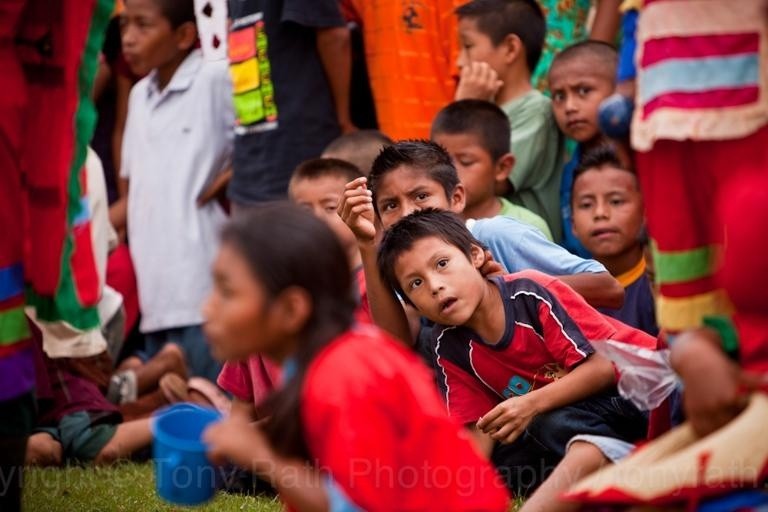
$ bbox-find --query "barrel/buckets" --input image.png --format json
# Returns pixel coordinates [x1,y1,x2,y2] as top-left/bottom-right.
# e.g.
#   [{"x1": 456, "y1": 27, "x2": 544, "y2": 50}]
[{"x1": 150, "y1": 401, "x2": 225, "y2": 506}]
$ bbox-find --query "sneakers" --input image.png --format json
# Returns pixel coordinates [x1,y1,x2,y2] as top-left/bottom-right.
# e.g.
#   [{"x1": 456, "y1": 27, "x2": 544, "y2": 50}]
[{"x1": 107, "y1": 373, "x2": 131, "y2": 406}]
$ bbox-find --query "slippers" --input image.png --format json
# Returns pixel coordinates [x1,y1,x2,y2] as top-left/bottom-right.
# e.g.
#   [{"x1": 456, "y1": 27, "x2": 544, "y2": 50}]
[{"x1": 159, "y1": 371, "x2": 235, "y2": 418}]
